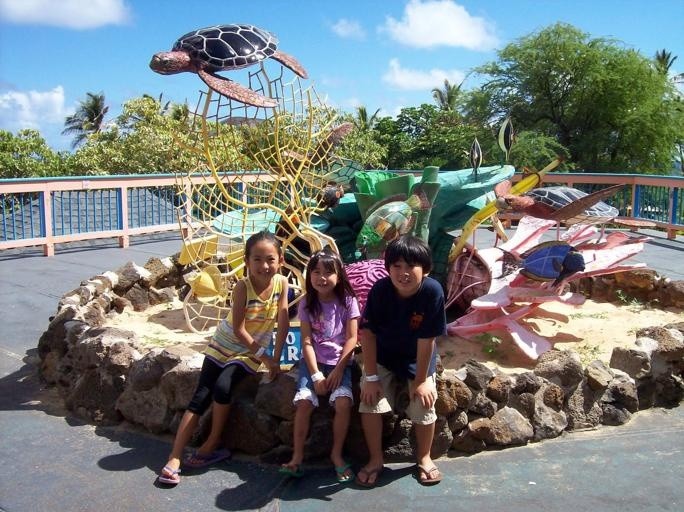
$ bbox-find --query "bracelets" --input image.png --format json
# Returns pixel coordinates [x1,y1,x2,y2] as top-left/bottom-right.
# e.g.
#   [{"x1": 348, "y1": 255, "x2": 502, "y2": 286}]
[
  {"x1": 255, "y1": 346, "x2": 266, "y2": 358},
  {"x1": 364, "y1": 374, "x2": 380, "y2": 383},
  {"x1": 310, "y1": 370, "x2": 325, "y2": 382}
]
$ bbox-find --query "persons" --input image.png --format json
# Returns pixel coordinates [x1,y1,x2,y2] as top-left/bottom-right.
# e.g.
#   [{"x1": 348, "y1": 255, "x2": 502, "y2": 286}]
[
  {"x1": 278, "y1": 251, "x2": 364, "y2": 483},
  {"x1": 356, "y1": 234, "x2": 448, "y2": 488},
  {"x1": 155, "y1": 232, "x2": 290, "y2": 483}
]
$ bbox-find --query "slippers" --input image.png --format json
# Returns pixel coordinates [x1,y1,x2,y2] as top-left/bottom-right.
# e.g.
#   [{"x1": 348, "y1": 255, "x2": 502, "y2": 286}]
[
  {"x1": 417, "y1": 465, "x2": 441, "y2": 483},
  {"x1": 159, "y1": 466, "x2": 181, "y2": 484},
  {"x1": 184, "y1": 448, "x2": 231, "y2": 467},
  {"x1": 335, "y1": 464, "x2": 383, "y2": 487},
  {"x1": 279, "y1": 464, "x2": 304, "y2": 477}
]
